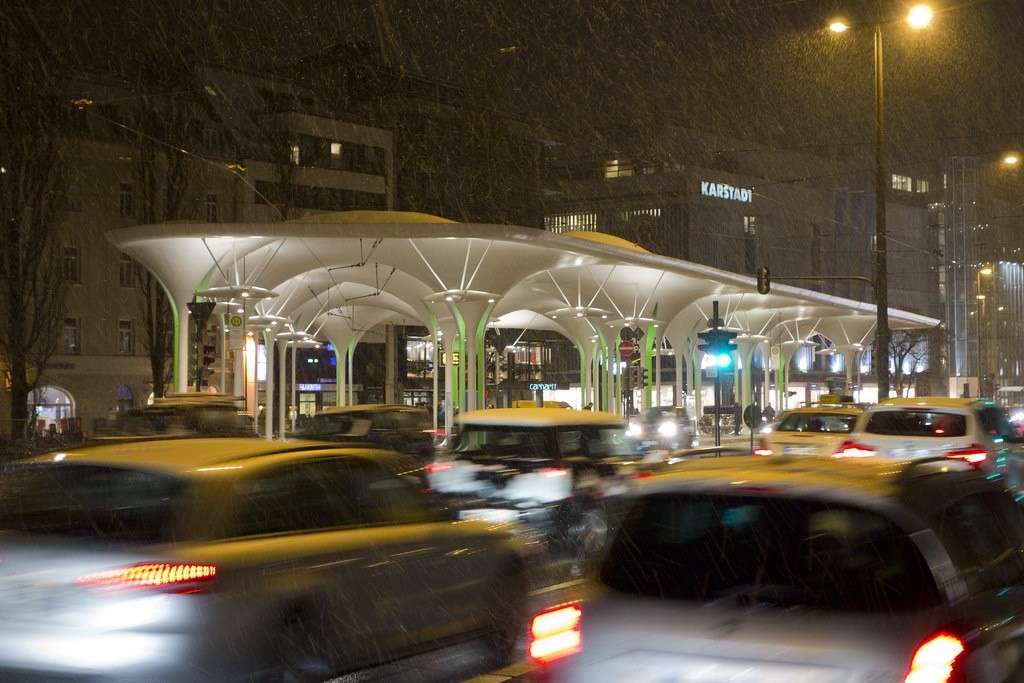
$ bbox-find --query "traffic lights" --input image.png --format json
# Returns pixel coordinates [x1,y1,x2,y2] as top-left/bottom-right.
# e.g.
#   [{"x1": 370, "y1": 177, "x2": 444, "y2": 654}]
[
  {"x1": 639, "y1": 367, "x2": 648, "y2": 388},
  {"x1": 199, "y1": 345, "x2": 216, "y2": 378},
  {"x1": 723, "y1": 331, "x2": 738, "y2": 351},
  {"x1": 716, "y1": 353, "x2": 732, "y2": 369},
  {"x1": 697, "y1": 332, "x2": 714, "y2": 354}
]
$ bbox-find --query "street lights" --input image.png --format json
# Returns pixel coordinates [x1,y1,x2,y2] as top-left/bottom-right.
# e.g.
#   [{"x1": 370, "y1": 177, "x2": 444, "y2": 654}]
[
  {"x1": 977, "y1": 265, "x2": 993, "y2": 398},
  {"x1": 827, "y1": 8, "x2": 935, "y2": 402}
]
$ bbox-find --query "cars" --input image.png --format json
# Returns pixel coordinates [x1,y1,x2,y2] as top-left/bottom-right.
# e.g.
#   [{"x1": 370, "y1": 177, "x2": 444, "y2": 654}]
[
  {"x1": 286, "y1": 404, "x2": 435, "y2": 459},
  {"x1": 524, "y1": 458, "x2": 1024, "y2": 683},
  {"x1": 828, "y1": 396, "x2": 1024, "y2": 494},
  {"x1": 627, "y1": 406, "x2": 693, "y2": 451},
  {"x1": 753, "y1": 394, "x2": 870, "y2": 458},
  {"x1": 113, "y1": 395, "x2": 259, "y2": 439},
  {"x1": 427, "y1": 406, "x2": 636, "y2": 527},
  {"x1": 0, "y1": 437, "x2": 548, "y2": 683}
]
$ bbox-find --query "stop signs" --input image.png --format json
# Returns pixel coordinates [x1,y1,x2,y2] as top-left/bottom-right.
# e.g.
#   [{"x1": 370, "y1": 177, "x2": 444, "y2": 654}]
[{"x1": 618, "y1": 341, "x2": 634, "y2": 356}]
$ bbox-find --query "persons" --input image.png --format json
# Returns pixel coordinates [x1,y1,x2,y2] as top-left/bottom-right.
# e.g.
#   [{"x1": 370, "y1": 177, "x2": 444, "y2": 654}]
[
  {"x1": 582, "y1": 401, "x2": 593, "y2": 411},
  {"x1": 734, "y1": 401, "x2": 776, "y2": 427},
  {"x1": 633, "y1": 407, "x2": 640, "y2": 415},
  {"x1": 415, "y1": 399, "x2": 495, "y2": 428}
]
